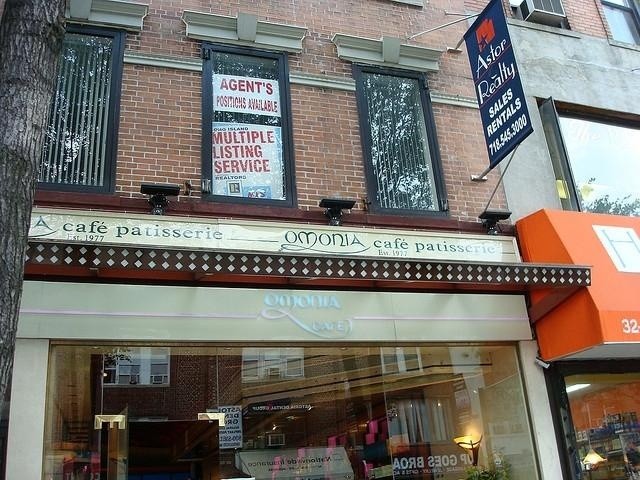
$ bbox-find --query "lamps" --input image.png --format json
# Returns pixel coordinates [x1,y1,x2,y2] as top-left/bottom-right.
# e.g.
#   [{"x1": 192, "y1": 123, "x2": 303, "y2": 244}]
[
  {"x1": 582, "y1": 415, "x2": 608, "y2": 468},
  {"x1": 456, "y1": 431, "x2": 487, "y2": 464},
  {"x1": 482, "y1": 210, "x2": 512, "y2": 236},
  {"x1": 141, "y1": 178, "x2": 182, "y2": 215},
  {"x1": 317, "y1": 195, "x2": 352, "y2": 225}
]
